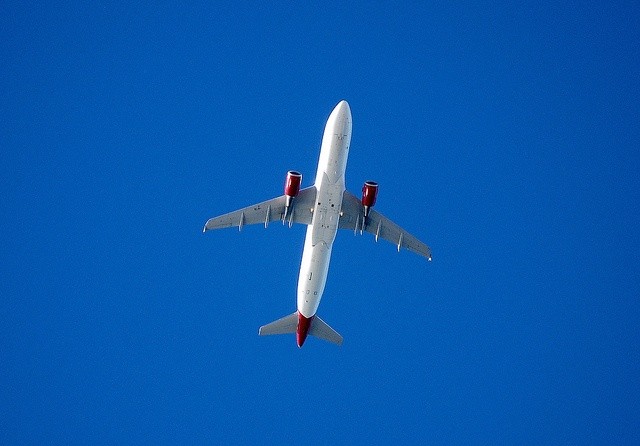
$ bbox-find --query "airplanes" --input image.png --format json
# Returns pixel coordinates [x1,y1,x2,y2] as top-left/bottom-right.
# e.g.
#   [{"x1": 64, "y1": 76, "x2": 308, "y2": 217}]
[{"x1": 203, "y1": 100, "x2": 431, "y2": 349}]
